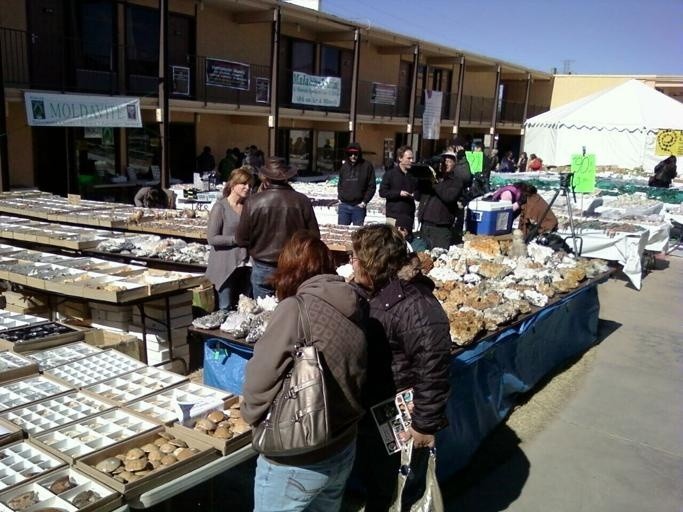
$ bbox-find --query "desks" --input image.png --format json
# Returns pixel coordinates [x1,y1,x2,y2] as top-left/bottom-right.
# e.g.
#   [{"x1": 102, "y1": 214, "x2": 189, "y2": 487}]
[{"x1": 1, "y1": 171, "x2": 682, "y2": 512}]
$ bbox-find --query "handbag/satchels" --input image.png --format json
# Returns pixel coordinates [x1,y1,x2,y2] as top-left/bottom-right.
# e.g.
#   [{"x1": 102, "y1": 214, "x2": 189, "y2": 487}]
[
  {"x1": 648, "y1": 176, "x2": 656, "y2": 187},
  {"x1": 459, "y1": 176, "x2": 485, "y2": 206},
  {"x1": 384, "y1": 435, "x2": 445, "y2": 512},
  {"x1": 249, "y1": 295, "x2": 332, "y2": 458},
  {"x1": 536, "y1": 232, "x2": 572, "y2": 254}
]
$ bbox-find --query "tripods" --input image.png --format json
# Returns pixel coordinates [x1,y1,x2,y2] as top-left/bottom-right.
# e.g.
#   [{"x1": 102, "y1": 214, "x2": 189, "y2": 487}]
[{"x1": 524, "y1": 173, "x2": 579, "y2": 258}]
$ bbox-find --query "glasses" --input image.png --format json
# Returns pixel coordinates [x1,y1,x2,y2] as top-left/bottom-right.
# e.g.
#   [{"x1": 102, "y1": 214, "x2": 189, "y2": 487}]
[
  {"x1": 347, "y1": 152, "x2": 358, "y2": 157},
  {"x1": 396, "y1": 227, "x2": 408, "y2": 231},
  {"x1": 348, "y1": 254, "x2": 359, "y2": 263}
]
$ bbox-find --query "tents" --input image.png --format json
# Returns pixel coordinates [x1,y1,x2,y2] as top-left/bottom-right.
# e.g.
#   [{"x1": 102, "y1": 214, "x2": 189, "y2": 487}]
[{"x1": 519, "y1": 79, "x2": 682, "y2": 170}]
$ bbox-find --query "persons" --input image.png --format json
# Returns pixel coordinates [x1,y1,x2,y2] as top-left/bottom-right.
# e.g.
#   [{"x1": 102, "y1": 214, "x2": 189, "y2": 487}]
[
  {"x1": 240, "y1": 232, "x2": 370, "y2": 512},
  {"x1": 347, "y1": 221, "x2": 455, "y2": 512},
  {"x1": 134, "y1": 186, "x2": 169, "y2": 208},
  {"x1": 652, "y1": 156, "x2": 677, "y2": 185}
]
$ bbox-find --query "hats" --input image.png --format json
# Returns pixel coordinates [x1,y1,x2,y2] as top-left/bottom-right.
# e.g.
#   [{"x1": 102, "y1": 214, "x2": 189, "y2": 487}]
[
  {"x1": 394, "y1": 213, "x2": 413, "y2": 230},
  {"x1": 258, "y1": 156, "x2": 297, "y2": 181},
  {"x1": 441, "y1": 150, "x2": 458, "y2": 163}
]
